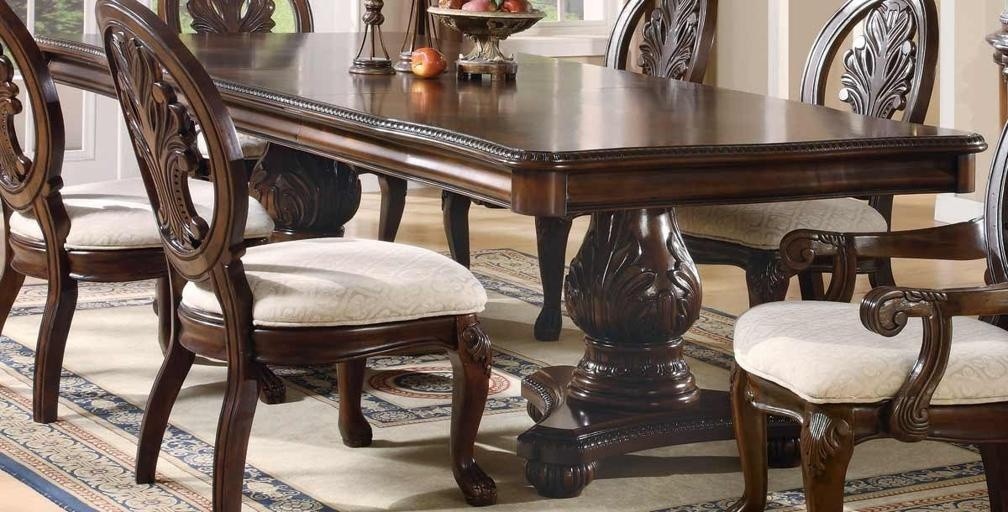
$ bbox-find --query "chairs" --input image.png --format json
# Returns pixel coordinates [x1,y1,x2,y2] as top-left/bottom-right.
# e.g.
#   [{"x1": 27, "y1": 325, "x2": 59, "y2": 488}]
[
  {"x1": 440, "y1": 1, "x2": 719, "y2": 342},
  {"x1": 731, "y1": 113, "x2": 1008, "y2": 512},
  {"x1": 156, "y1": 0, "x2": 407, "y2": 241},
  {"x1": 96, "y1": 0, "x2": 498, "y2": 512},
  {"x1": 675, "y1": 1, "x2": 940, "y2": 314},
  {"x1": 0, "y1": 0, "x2": 286, "y2": 424}
]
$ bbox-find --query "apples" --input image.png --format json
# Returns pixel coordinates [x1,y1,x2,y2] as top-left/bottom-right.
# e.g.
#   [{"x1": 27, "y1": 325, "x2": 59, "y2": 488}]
[
  {"x1": 412, "y1": 79, "x2": 444, "y2": 107},
  {"x1": 501, "y1": 0, "x2": 530, "y2": 13},
  {"x1": 461, "y1": 1, "x2": 496, "y2": 11},
  {"x1": 438, "y1": 0, "x2": 462, "y2": 9},
  {"x1": 411, "y1": 48, "x2": 448, "y2": 78}
]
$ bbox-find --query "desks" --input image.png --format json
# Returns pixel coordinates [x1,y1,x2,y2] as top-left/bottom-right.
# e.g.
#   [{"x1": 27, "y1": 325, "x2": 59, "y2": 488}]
[{"x1": 28, "y1": 30, "x2": 988, "y2": 498}]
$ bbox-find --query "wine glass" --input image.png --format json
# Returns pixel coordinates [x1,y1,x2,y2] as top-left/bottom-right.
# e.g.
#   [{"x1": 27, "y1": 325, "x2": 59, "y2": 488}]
[{"x1": 426, "y1": 5, "x2": 546, "y2": 81}]
